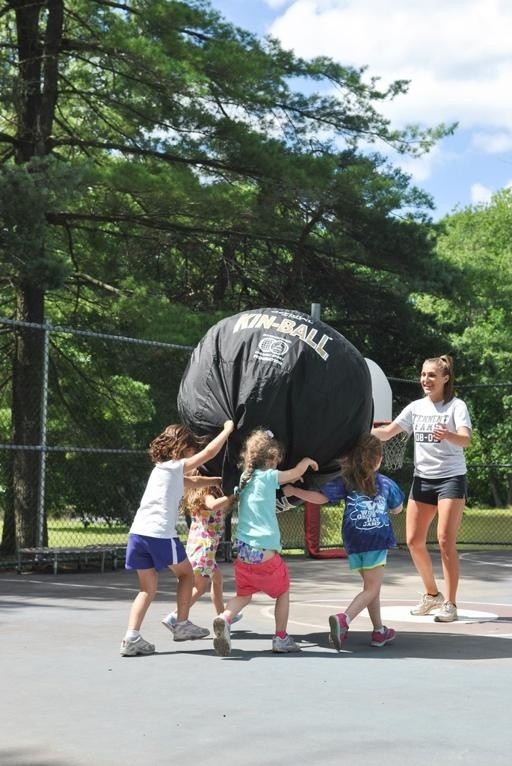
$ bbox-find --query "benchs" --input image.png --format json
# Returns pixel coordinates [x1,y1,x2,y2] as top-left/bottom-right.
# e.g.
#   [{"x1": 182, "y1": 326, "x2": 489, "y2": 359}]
[{"x1": 16, "y1": 547, "x2": 125, "y2": 576}]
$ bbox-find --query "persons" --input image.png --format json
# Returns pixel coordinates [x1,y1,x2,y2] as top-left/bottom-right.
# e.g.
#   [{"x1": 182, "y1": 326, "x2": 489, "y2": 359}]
[
  {"x1": 370, "y1": 355, "x2": 473, "y2": 624},
  {"x1": 211, "y1": 426, "x2": 320, "y2": 660},
  {"x1": 161, "y1": 465, "x2": 243, "y2": 635},
  {"x1": 280, "y1": 432, "x2": 405, "y2": 648},
  {"x1": 118, "y1": 418, "x2": 236, "y2": 657}
]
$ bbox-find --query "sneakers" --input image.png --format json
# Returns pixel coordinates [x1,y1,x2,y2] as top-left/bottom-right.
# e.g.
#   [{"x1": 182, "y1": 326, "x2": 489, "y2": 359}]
[
  {"x1": 372, "y1": 625, "x2": 396, "y2": 646},
  {"x1": 162, "y1": 612, "x2": 178, "y2": 633},
  {"x1": 329, "y1": 613, "x2": 349, "y2": 652},
  {"x1": 231, "y1": 613, "x2": 243, "y2": 624},
  {"x1": 172, "y1": 620, "x2": 211, "y2": 640},
  {"x1": 434, "y1": 602, "x2": 458, "y2": 622},
  {"x1": 119, "y1": 634, "x2": 156, "y2": 657},
  {"x1": 410, "y1": 592, "x2": 446, "y2": 616},
  {"x1": 273, "y1": 633, "x2": 302, "y2": 654},
  {"x1": 213, "y1": 616, "x2": 231, "y2": 657}
]
